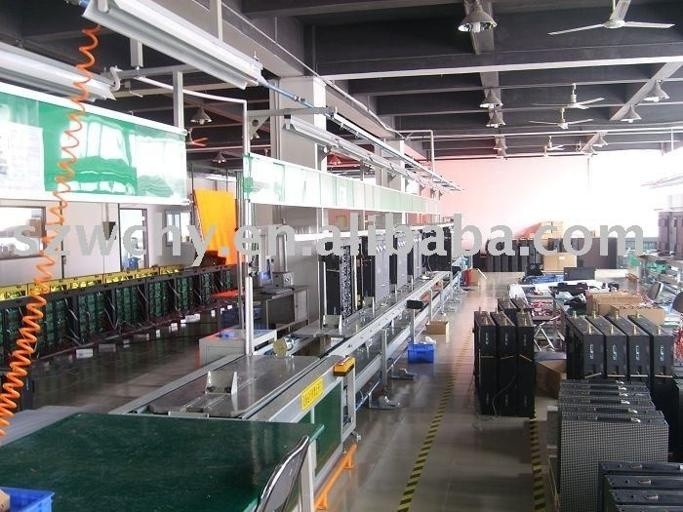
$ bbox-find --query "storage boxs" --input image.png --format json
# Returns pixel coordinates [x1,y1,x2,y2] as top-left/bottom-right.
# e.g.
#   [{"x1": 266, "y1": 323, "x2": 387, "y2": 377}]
[
  {"x1": 611, "y1": 305, "x2": 666, "y2": 324},
  {"x1": 535, "y1": 359, "x2": 567, "y2": 401},
  {"x1": 425, "y1": 318, "x2": 450, "y2": 335},
  {"x1": 0, "y1": 487, "x2": 54, "y2": 512},
  {"x1": 408, "y1": 344, "x2": 434, "y2": 363},
  {"x1": 542, "y1": 252, "x2": 577, "y2": 271},
  {"x1": 27, "y1": 219, "x2": 43, "y2": 238},
  {"x1": 587, "y1": 293, "x2": 642, "y2": 315},
  {"x1": 462, "y1": 267, "x2": 481, "y2": 287}
]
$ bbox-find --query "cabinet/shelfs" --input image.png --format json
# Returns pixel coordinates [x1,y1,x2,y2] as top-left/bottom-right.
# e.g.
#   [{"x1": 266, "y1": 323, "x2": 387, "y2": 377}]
[
  {"x1": 200, "y1": 329, "x2": 277, "y2": 365},
  {"x1": 198, "y1": 284, "x2": 306, "y2": 332}
]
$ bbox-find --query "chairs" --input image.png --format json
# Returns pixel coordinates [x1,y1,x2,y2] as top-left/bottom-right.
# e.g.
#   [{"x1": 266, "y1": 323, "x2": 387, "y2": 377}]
[{"x1": 254, "y1": 434, "x2": 310, "y2": 511}]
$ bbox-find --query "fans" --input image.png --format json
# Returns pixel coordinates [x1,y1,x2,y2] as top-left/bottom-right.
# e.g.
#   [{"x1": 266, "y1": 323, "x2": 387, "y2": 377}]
[
  {"x1": 532, "y1": 96, "x2": 605, "y2": 110},
  {"x1": 547, "y1": 144, "x2": 566, "y2": 151},
  {"x1": 528, "y1": 118, "x2": 594, "y2": 130},
  {"x1": 187, "y1": 130, "x2": 207, "y2": 148},
  {"x1": 546, "y1": 0, "x2": 676, "y2": 37}
]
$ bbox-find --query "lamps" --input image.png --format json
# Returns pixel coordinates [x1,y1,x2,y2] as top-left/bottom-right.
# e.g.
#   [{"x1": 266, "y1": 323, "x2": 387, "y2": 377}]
[
  {"x1": 492, "y1": 139, "x2": 508, "y2": 151},
  {"x1": 479, "y1": 88, "x2": 504, "y2": 110},
  {"x1": 279, "y1": 115, "x2": 339, "y2": 156},
  {"x1": 338, "y1": 133, "x2": 368, "y2": 167},
  {"x1": 368, "y1": 150, "x2": 392, "y2": 172},
  {"x1": 485, "y1": 110, "x2": 507, "y2": 129},
  {"x1": 643, "y1": 80, "x2": 670, "y2": 103},
  {"x1": 212, "y1": 152, "x2": 228, "y2": 164},
  {"x1": 496, "y1": 150, "x2": 508, "y2": 160},
  {"x1": 582, "y1": 145, "x2": 598, "y2": 158},
  {"x1": 592, "y1": 134, "x2": 608, "y2": 148},
  {"x1": 81, "y1": 0, "x2": 264, "y2": 91},
  {"x1": 458, "y1": 0, "x2": 497, "y2": 34},
  {"x1": 394, "y1": 162, "x2": 450, "y2": 198},
  {"x1": 620, "y1": 104, "x2": 643, "y2": 124},
  {"x1": 190, "y1": 106, "x2": 212, "y2": 125}
]
